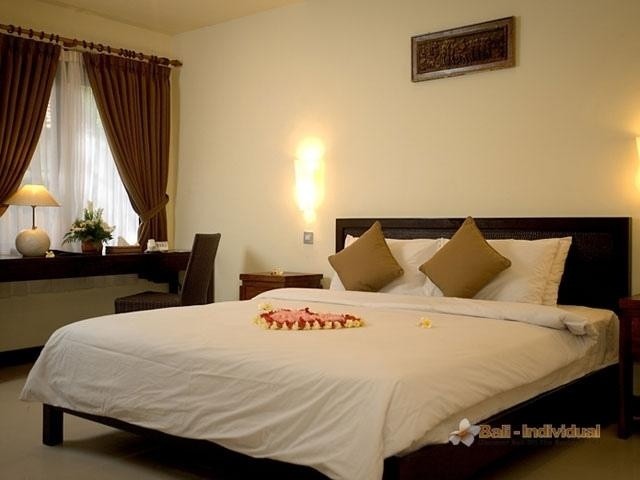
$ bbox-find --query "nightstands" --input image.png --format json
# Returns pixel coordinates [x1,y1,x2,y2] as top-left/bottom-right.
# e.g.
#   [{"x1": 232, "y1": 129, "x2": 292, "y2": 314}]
[{"x1": 236, "y1": 269, "x2": 324, "y2": 304}]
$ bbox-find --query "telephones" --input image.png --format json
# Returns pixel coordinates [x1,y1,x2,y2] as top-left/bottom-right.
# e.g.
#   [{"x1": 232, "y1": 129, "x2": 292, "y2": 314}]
[{"x1": 147, "y1": 239, "x2": 169, "y2": 252}]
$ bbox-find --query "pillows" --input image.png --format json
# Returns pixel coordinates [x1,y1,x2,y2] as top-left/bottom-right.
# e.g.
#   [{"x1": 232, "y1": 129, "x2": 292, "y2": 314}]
[
  {"x1": 327, "y1": 220, "x2": 404, "y2": 294},
  {"x1": 328, "y1": 234, "x2": 438, "y2": 298},
  {"x1": 427, "y1": 236, "x2": 574, "y2": 308},
  {"x1": 417, "y1": 215, "x2": 513, "y2": 299}
]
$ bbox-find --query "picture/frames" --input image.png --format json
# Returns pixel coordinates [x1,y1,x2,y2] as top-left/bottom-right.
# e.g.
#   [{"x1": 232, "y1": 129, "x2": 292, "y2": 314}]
[{"x1": 409, "y1": 16, "x2": 518, "y2": 85}]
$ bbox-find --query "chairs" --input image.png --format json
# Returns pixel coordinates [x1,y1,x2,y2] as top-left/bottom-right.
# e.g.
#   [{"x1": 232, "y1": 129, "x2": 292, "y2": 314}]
[{"x1": 114, "y1": 232, "x2": 224, "y2": 314}]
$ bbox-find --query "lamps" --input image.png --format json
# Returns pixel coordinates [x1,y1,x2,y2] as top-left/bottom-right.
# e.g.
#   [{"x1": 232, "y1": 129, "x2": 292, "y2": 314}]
[{"x1": 1, "y1": 182, "x2": 62, "y2": 257}]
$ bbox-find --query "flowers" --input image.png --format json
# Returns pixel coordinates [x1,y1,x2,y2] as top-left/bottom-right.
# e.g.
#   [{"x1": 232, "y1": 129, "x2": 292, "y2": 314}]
[
  {"x1": 413, "y1": 315, "x2": 433, "y2": 330},
  {"x1": 253, "y1": 303, "x2": 367, "y2": 332},
  {"x1": 62, "y1": 198, "x2": 118, "y2": 245}
]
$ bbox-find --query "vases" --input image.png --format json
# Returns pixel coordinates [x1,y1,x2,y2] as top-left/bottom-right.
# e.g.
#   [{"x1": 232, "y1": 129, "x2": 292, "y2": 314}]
[{"x1": 81, "y1": 238, "x2": 104, "y2": 254}]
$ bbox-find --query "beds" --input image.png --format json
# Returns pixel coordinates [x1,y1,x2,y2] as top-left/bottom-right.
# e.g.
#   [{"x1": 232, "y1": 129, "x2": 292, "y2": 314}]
[{"x1": 19, "y1": 211, "x2": 639, "y2": 480}]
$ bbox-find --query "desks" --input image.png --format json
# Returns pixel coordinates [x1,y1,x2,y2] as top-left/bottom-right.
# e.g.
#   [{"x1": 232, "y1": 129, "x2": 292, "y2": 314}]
[
  {"x1": 616, "y1": 288, "x2": 640, "y2": 438},
  {"x1": 0, "y1": 247, "x2": 217, "y2": 330}
]
